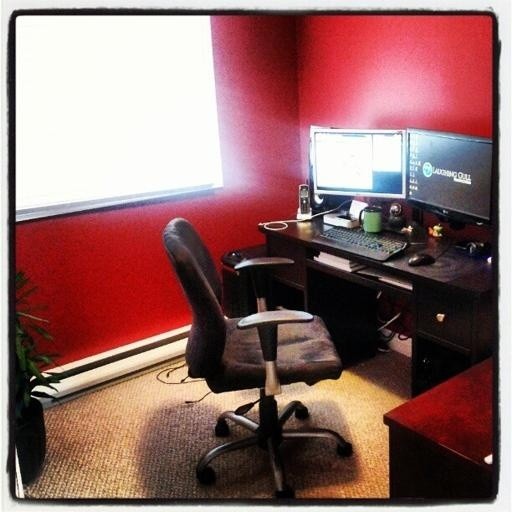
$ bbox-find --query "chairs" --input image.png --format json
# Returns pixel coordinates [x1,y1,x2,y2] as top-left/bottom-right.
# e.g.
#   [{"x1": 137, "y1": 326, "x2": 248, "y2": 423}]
[{"x1": 161, "y1": 217, "x2": 353, "y2": 499}]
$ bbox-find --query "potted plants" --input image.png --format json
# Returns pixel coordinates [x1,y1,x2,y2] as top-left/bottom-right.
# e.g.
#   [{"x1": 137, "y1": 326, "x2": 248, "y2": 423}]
[{"x1": 13, "y1": 271, "x2": 66, "y2": 485}]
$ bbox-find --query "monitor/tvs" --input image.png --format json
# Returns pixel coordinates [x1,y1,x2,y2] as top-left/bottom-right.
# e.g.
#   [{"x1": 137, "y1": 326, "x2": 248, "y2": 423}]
[
  {"x1": 313, "y1": 129, "x2": 406, "y2": 199},
  {"x1": 406, "y1": 128, "x2": 493, "y2": 224}
]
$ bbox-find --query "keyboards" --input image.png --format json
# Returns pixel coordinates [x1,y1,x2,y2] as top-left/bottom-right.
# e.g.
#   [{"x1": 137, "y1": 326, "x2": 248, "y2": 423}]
[{"x1": 312, "y1": 226, "x2": 408, "y2": 261}]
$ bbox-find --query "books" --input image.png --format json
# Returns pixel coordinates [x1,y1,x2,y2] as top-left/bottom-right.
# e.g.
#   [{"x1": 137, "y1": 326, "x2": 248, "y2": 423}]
[{"x1": 313, "y1": 251, "x2": 368, "y2": 273}]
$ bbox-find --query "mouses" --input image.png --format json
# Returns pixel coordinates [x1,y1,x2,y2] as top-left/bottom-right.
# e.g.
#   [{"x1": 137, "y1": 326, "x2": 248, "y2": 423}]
[{"x1": 409, "y1": 253, "x2": 435, "y2": 267}]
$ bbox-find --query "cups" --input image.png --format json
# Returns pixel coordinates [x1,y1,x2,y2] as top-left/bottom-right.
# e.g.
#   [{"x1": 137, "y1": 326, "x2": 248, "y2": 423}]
[{"x1": 359, "y1": 206, "x2": 384, "y2": 233}]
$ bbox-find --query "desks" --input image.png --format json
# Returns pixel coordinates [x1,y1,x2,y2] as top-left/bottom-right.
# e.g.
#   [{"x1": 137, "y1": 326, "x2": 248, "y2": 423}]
[{"x1": 258, "y1": 218, "x2": 493, "y2": 398}]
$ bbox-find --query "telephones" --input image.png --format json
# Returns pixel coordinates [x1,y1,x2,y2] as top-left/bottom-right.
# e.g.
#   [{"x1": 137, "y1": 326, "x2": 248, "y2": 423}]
[{"x1": 296, "y1": 184, "x2": 312, "y2": 220}]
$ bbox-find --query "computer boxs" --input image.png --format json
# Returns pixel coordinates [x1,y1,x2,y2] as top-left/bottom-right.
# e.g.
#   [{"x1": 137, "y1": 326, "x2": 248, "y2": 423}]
[{"x1": 220, "y1": 244, "x2": 275, "y2": 318}]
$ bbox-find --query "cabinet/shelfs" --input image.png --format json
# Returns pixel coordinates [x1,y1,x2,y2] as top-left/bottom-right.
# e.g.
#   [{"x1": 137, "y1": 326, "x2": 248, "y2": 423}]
[{"x1": 383, "y1": 356, "x2": 493, "y2": 498}]
